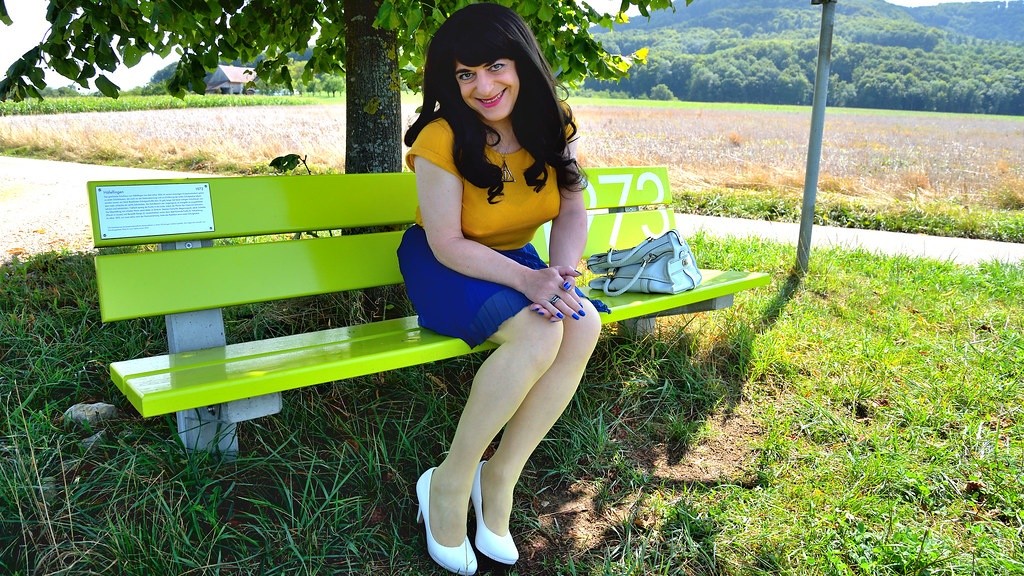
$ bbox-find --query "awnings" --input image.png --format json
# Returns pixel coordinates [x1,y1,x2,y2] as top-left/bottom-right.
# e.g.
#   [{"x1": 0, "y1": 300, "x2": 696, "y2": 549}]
[{"x1": 207, "y1": 80, "x2": 228, "y2": 89}]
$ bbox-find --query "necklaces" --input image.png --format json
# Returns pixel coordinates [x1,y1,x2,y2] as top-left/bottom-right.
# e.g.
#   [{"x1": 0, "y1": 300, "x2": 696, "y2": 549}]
[{"x1": 491, "y1": 132, "x2": 516, "y2": 182}]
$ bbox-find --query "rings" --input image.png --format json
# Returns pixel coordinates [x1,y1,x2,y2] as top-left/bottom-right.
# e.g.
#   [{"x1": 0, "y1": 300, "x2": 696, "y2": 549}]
[{"x1": 550, "y1": 295, "x2": 559, "y2": 306}]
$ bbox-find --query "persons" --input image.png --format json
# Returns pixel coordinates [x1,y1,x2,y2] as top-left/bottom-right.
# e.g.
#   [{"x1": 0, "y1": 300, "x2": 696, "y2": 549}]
[{"x1": 397, "y1": 2, "x2": 610, "y2": 574}]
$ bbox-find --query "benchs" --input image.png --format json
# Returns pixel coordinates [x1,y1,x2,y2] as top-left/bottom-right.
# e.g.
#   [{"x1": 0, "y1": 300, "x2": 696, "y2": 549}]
[{"x1": 87, "y1": 167, "x2": 769, "y2": 507}]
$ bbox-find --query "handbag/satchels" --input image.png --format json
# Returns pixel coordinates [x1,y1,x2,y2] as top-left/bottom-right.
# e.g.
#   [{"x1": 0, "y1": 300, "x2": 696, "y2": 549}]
[{"x1": 586, "y1": 229, "x2": 701, "y2": 297}]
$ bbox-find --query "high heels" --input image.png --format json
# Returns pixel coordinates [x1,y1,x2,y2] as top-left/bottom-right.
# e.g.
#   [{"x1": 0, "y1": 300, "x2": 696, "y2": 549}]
[
  {"x1": 466, "y1": 460, "x2": 519, "y2": 565},
  {"x1": 416, "y1": 468, "x2": 478, "y2": 576}
]
